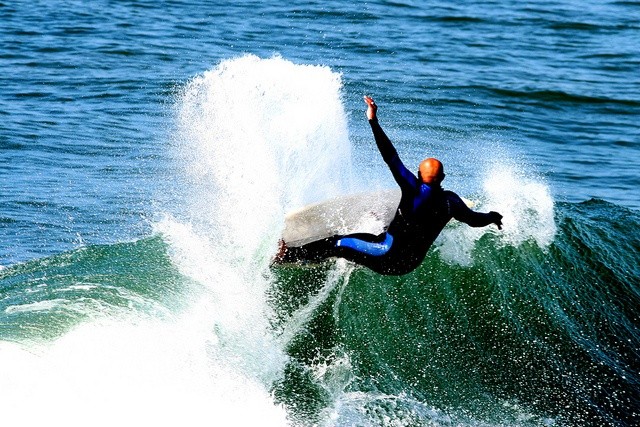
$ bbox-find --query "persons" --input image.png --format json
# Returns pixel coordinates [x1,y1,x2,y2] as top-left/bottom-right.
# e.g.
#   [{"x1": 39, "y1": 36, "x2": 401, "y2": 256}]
[{"x1": 277, "y1": 95, "x2": 504, "y2": 276}]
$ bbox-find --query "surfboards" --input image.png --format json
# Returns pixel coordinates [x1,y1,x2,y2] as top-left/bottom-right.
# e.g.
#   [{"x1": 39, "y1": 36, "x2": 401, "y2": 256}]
[{"x1": 279, "y1": 191, "x2": 479, "y2": 246}]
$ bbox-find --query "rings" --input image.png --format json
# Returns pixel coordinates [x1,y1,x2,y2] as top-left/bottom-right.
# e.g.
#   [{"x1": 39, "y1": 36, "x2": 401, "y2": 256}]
[{"x1": 371, "y1": 102, "x2": 375, "y2": 105}]
